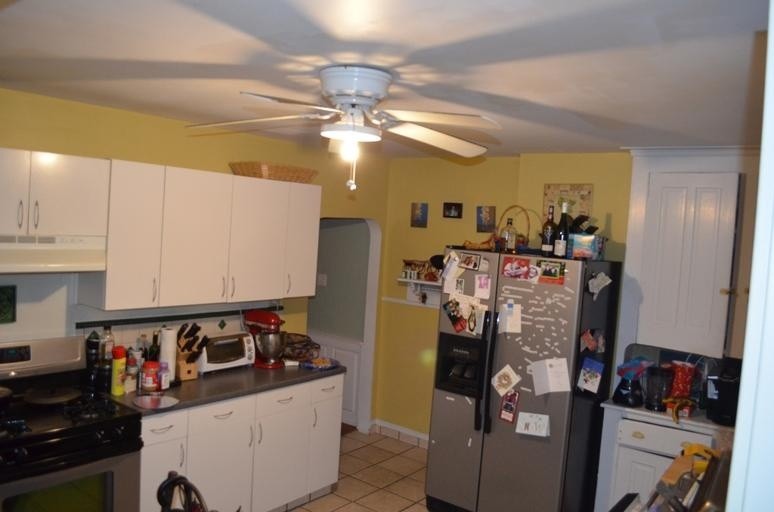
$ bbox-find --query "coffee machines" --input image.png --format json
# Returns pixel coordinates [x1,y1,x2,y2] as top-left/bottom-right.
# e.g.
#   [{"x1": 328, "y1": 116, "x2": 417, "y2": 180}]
[{"x1": 242, "y1": 310, "x2": 287, "y2": 369}]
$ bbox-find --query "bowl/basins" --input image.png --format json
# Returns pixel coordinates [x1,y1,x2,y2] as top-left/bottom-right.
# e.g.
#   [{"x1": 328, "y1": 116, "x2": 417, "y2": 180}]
[{"x1": 281, "y1": 333, "x2": 319, "y2": 361}]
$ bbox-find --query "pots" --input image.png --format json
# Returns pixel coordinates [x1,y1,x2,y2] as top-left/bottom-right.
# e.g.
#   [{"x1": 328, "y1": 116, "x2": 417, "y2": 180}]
[{"x1": 23, "y1": 388, "x2": 82, "y2": 408}]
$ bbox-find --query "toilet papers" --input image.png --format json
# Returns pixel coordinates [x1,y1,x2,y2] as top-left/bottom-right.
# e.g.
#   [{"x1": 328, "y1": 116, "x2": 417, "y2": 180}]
[{"x1": 158, "y1": 327, "x2": 178, "y2": 383}]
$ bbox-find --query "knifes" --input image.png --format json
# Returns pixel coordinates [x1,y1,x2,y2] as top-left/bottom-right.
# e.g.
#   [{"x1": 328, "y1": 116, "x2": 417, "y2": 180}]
[{"x1": 176, "y1": 321, "x2": 207, "y2": 363}]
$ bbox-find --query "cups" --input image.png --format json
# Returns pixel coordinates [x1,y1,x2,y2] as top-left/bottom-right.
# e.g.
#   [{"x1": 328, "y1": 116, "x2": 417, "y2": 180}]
[
  {"x1": 401, "y1": 270, "x2": 417, "y2": 280},
  {"x1": 643, "y1": 361, "x2": 695, "y2": 411}
]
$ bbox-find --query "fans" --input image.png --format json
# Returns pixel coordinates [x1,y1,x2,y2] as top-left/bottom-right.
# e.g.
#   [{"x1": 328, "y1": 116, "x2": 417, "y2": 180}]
[{"x1": 184, "y1": 63, "x2": 489, "y2": 193}]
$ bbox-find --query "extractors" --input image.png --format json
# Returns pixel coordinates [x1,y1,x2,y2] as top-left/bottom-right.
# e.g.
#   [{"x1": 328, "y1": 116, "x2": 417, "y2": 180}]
[{"x1": 0, "y1": 236, "x2": 106, "y2": 273}]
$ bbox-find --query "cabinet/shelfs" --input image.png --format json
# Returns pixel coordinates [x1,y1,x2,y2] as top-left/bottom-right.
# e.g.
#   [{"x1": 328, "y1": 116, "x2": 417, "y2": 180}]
[
  {"x1": 284, "y1": 178, "x2": 323, "y2": 300},
  {"x1": 307, "y1": 373, "x2": 345, "y2": 501},
  {"x1": 593, "y1": 398, "x2": 734, "y2": 512},
  {"x1": 114, "y1": 408, "x2": 189, "y2": 511},
  {"x1": 619, "y1": 146, "x2": 761, "y2": 362},
  {"x1": 187, "y1": 383, "x2": 310, "y2": 512},
  {"x1": 159, "y1": 166, "x2": 284, "y2": 308},
  {"x1": 1, "y1": 147, "x2": 110, "y2": 251},
  {"x1": 78, "y1": 158, "x2": 167, "y2": 313}
]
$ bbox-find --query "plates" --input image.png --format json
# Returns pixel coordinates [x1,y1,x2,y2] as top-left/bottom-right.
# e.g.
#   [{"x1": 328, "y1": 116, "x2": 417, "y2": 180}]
[{"x1": 305, "y1": 358, "x2": 339, "y2": 368}]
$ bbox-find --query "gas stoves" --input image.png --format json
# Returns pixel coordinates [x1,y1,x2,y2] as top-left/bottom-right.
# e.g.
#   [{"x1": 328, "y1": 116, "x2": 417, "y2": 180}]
[{"x1": 0, "y1": 386, "x2": 118, "y2": 434}]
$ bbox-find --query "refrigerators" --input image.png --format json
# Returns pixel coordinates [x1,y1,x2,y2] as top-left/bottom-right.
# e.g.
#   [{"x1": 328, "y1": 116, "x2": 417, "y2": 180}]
[{"x1": 424, "y1": 245, "x2": 622, "y2": 511}]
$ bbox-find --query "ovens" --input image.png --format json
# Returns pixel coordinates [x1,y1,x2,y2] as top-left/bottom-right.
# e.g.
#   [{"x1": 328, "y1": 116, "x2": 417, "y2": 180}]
[{"x1": 0, "y1": 451, "x2": 141, "y2": 511}]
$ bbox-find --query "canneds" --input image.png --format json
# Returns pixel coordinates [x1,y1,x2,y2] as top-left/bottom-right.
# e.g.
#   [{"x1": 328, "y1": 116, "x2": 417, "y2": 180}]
[
  {"x1": 157, "y1": 362, "x2": 171, "y2": 389},
  {"x1": 88, "y1": 339, "x2": 100, "y2": 365},
  {"x1": 141, "y1": 361, "x2": 159, "y2": 391}
]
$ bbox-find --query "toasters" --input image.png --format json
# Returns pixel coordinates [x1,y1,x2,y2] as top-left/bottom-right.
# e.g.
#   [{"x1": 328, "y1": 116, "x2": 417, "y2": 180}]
[{"x1": 197, "y1": 331, "x2": 256, "y2": 375}]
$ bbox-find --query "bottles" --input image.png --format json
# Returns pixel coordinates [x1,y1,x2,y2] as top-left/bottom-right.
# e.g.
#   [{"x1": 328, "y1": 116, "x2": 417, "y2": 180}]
[
  {"x1": 541, "y1": 201, "x2": 569, "y2": 258},
  {"x1": 86, "y1": 324, "x2": 176, "y2": 396},
  {"x1": 498, "y1": 218, "x2": 518, "y2": 254},
  {"x1": 568, "y1": 216, "x2": 588, "y2": 234}
]
